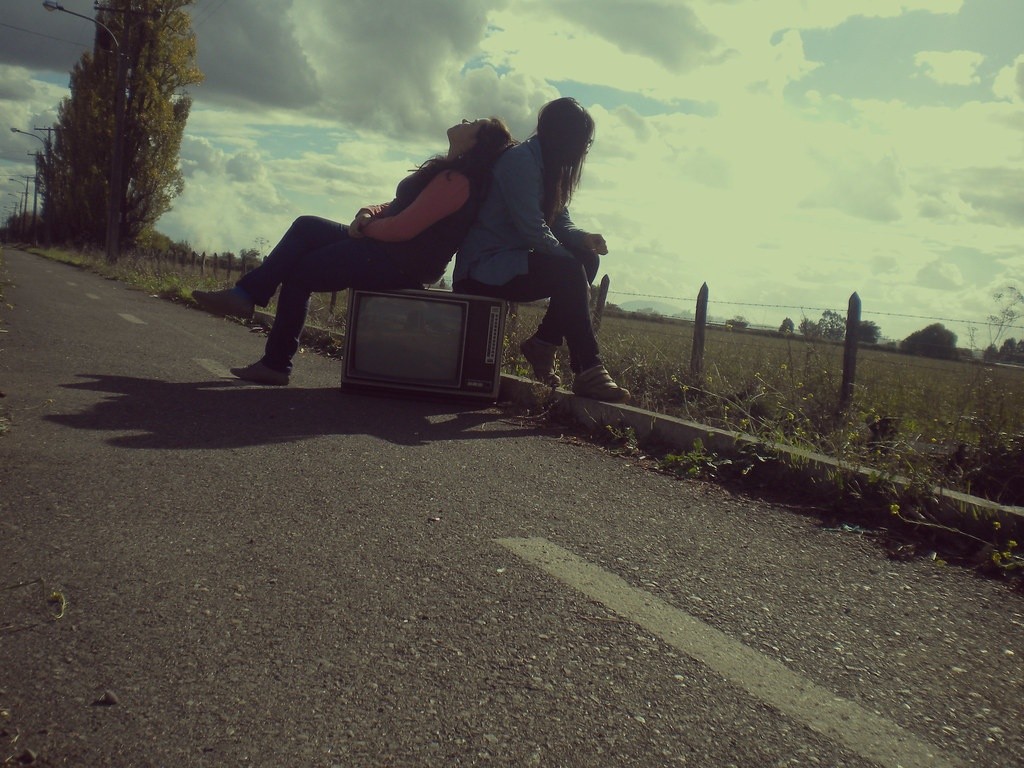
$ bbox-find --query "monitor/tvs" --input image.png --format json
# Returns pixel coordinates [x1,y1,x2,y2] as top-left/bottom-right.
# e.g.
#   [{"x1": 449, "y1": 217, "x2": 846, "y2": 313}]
[{"x1": 340, "y1": 285, "x2": 508, "y2": 405}]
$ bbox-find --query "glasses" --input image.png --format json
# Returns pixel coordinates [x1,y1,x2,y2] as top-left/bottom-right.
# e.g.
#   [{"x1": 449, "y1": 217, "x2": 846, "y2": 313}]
[{"x1": 582, "y1": 137, "x2": 593, "y2": 154}]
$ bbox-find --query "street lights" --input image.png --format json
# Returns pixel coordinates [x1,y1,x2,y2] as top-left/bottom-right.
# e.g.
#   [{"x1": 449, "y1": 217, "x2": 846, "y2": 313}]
[
  {"x1": 7, "y1": 175, "x2": 29, "y2": 246},
  {"x1": 8, "y1": 126, "x2": 50, "y2": 250},
  {"x1": 42, "y1": 0, "x2": 131, "y2": 265},
  {"x1": 8, "y1": 193, "x2": 24, "y2": 242}
]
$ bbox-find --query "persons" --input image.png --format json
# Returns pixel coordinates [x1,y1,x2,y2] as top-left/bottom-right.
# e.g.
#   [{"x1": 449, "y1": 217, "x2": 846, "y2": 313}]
[
  {"x1": 452, "y1": 97, "x2": 631, "y2": 404},
  {"x1": 192, "y1": 117, "x2": 523, "y2": 385}
]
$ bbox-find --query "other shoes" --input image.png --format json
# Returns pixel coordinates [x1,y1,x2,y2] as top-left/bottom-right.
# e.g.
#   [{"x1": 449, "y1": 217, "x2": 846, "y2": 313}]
[
  {"x1": 520, "y1": 336, "x2": 561, "y2": 386},
  {"x1": 191, "y1": 290, "x2": 254, "y2": 319},
  {"x1": 231, "y1": 360, "x2": 290, "y2": 385},
  {"x1": 572, "y1": 365, "x2": 631, "y2": 403}
]
